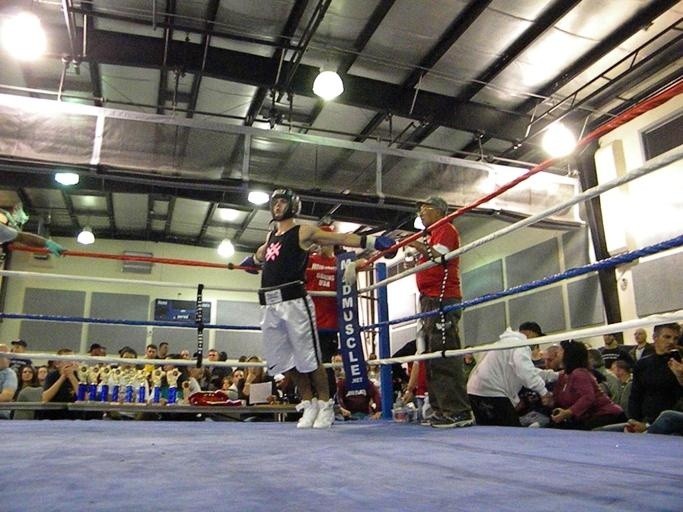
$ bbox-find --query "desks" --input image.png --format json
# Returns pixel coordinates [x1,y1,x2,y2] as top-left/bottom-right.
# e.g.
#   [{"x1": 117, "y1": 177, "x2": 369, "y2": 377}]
[
  {"x1": 0, "y1": 396, "x2": 76, "y2": 421},
  {"x1": 66, "y1": 398, "x2": 308, "y2": 424}
]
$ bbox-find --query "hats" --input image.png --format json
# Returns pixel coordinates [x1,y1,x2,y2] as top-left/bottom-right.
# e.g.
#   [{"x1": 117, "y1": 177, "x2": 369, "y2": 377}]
[
  {"x1": 10, "y1": 338, "x2": 27, "y2": 346},
  {"x1": 87, "y1": 343, "x2": 106, "y2": 353},
  {"x1": 519, "y1": 321, "x2": 547, "y2": 338},
  {"x1": 416, "y1": 195, "x2": 449, "y2": 214}
]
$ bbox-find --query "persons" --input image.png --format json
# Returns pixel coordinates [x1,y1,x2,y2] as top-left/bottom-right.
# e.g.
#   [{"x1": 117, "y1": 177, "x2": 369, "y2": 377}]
[
  {"x1": 304, "y1": 213, "x2": 372, "y2": 420},
  {"x1": 399, "y1": 197, "x2": 477, "y2": 427},
  {"x1": 392, "y1": 321, "x2": 683, "y2": 437},
  {"x1": 239, "y1": 186, "x2": 394, "y2": 429},
  {"x1": 0, "y1": 220, "x2": 67, "y2": 260}
]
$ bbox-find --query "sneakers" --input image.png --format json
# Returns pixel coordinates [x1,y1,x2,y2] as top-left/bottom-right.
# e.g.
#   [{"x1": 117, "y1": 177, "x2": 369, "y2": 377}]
[
  {"x1": 314, "y1": 398, "x2": 336, "y2": 429},
  {"x1": 294, "y1": 397, "x2": 319, "y2": 429},
  {"x1": 420, "y1": 410, "x2": 478, "y2": 429}
]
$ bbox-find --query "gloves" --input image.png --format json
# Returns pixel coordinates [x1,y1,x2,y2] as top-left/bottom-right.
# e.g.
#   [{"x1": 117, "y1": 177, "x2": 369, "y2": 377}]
[
  {"x1": 232, "y1": 255, "x2": 263, "y2": 273},
  {"x1": 361, "y1": 234, "x2": 399, "y2": 261}
]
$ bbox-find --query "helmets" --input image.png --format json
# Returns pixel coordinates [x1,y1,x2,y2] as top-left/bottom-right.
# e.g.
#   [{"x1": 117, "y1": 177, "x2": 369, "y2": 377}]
[{"x1": 270, "y1": 186, "x2": 301, "y2": 219}]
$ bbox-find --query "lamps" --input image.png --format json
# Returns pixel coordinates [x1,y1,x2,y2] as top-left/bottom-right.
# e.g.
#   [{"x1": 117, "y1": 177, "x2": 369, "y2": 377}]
[
  {"x1": 309, "y1": 42, "x2": 345, "y2": 102},
  {"x1": 216, "y1": 222, "x2": 236, "y2": 259},
  {"x1": 75, "y1": 210, "x2": 98, "y2": 248}
]
